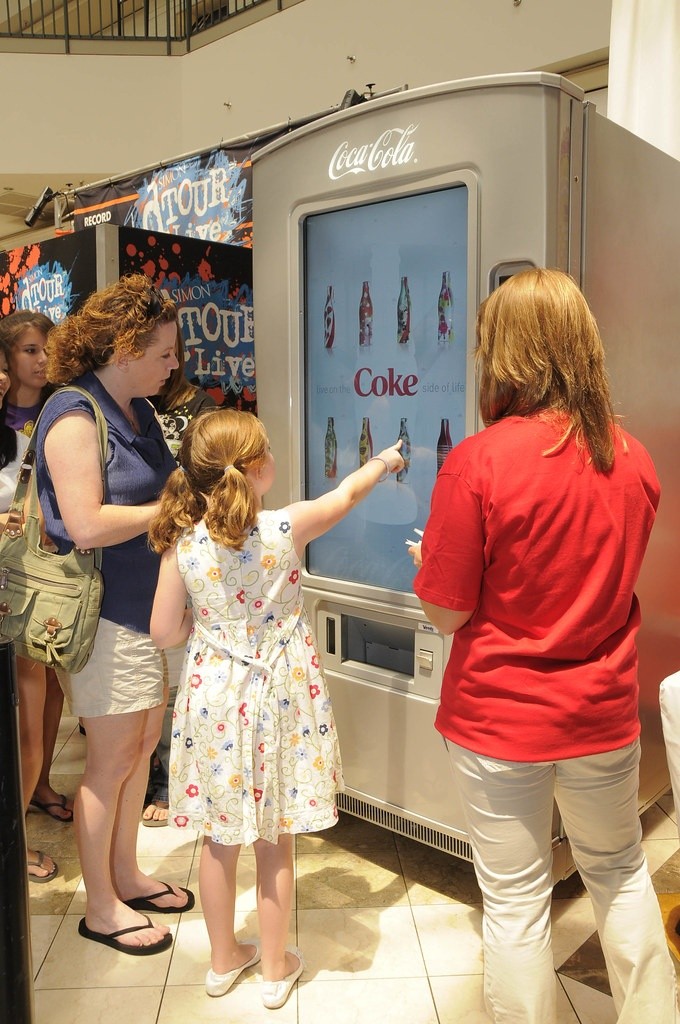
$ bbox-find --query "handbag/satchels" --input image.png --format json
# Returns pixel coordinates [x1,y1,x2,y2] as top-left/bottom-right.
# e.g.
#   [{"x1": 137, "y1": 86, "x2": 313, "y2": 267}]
[{"x1": 0, "y1": 386, "x2": 109, "y2": 673}]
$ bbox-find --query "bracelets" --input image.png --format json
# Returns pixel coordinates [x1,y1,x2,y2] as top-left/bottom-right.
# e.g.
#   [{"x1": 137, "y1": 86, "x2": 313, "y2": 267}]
[{"x1": 368, "y1": 456, "x2": 391, "y2": 482}]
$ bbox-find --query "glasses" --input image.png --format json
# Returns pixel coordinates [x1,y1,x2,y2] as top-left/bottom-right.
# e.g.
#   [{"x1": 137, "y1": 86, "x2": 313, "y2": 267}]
[{"x1": 140, "y1": 285, "x2": 163, "y2": 328}]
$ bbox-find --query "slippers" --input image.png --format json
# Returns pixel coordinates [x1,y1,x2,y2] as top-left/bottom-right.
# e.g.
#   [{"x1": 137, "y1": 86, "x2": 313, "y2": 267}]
[
  {"x1": 28, "y1": 850, "x2": 59, "y2": 884},
  {"x1": 143, "y1": 802, "x2": 169, "y2": 827},
  {"x1": 30, "y1": 794, "x2": 74, "y2": 822},
  {"x1": 79, "y1": 914, "x2": 172, "y2": 955},
  {"x1": 124, "y1": 881, "x2": 194, "y2": 913}
]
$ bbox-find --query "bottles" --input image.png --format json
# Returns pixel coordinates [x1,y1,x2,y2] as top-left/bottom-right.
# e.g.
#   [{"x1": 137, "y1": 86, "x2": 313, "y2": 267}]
[
  {"x1": 359, "y1": 417, "x2": 373, "y2": 468},
  {"x1": 396, "y1": 418, "x2": 412, "y2": 483},
  {"x1": 437, "y1": 418, "x2": 452, "y2": 476},
  {"x1": 324, "y1": 417, "x2": 337, "y2": 478},
  {"x1": 323, "y1": 285, "x2": 336, "y2": 348},
  {"x1": 397, "y1": 276, "x2": 411, "y2": 344},
  {"x1": 358, "y1": 281, "x2": 373, "y2": 346},
  {"x1": 437, "y1": 271, "x2": 454, "y2": 344}
]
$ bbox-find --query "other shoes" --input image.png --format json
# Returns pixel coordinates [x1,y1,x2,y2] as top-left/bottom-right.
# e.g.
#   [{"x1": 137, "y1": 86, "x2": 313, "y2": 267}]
[
  {"x1": 206, "y1": 939, "x2": 263, "y2": 996},
  {"x1": 262, "y1": 945, "x2": 303, "y2": 1009}
]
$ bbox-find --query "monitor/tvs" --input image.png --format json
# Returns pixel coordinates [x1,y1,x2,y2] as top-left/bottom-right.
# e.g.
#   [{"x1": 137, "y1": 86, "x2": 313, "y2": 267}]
[{"x1": 301, "y1": 184, "x2": 468, "y2": 596}]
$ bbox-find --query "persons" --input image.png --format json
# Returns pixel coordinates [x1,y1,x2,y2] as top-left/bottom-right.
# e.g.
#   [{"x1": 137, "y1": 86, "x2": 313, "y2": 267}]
[
  {"x1": 147, "y1": 409, "x2": 407, "y2": 1010},
  {"x1": 29, "y1": 272, "x2": 196, "y2": 955},
  {"x1": 0, "y1": 309, "x2": 76, "y2": 887},
  {"x1": 147, "y1": 322, "x2": 216, "y2": 466},
  {"x1": 407, "y1": 271, "x2": 679, "y2": 1024}
]
